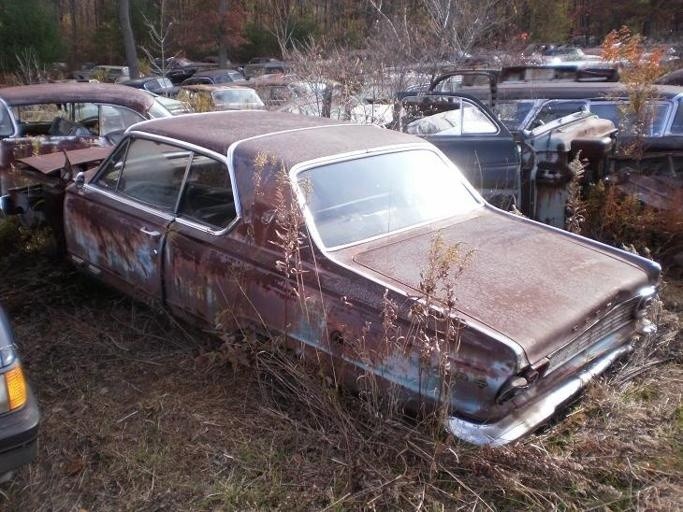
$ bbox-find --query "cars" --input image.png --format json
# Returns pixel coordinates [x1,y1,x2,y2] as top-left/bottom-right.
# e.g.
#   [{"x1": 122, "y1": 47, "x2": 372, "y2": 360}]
[
  {"x1": 0, "y1": 110, "x2": 663, "y2": 452},
  {"x1": 0, "y1": 307, "x2": 39, "y2": 479}
]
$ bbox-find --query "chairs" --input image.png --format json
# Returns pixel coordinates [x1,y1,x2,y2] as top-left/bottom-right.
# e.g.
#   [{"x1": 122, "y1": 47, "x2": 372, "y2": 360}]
[
  {"x1": 45, "y1": 117, "x2": 88, "y2": 138},
  {"x1": 179, "y1": 184, "x2": 236, "y2": 227}
]
